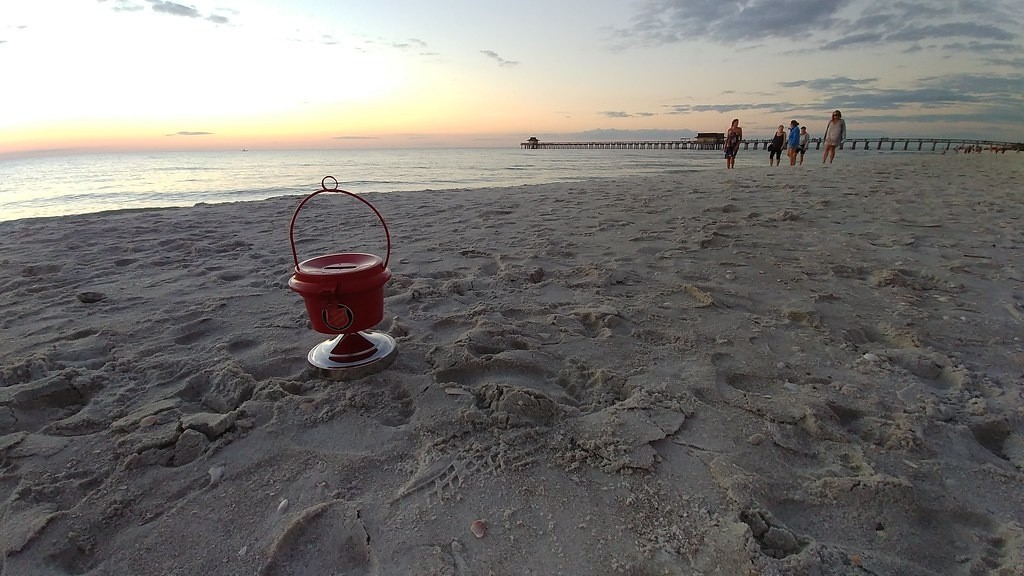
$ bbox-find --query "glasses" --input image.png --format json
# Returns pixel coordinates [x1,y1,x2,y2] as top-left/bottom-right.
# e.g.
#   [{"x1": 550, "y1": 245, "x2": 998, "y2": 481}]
[{"x1": 833, "y1": 114, "x2": 838, "y2": 116}]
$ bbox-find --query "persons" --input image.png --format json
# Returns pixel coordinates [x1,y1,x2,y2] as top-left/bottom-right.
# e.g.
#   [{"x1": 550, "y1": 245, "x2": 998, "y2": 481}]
[
  {"x1": 724, "y1": 119, "x2": 743, "y2": 169},
  {"x1": 786, "y1": 120, "x2": 800, "y2": 167},
  {"x1": 792, "y1": 126, "x2": 810, "y2": 166},
  {"x1": 822, "y1": 109, "x2": 847, "y2": 164},
  {"x1": 769, "y1": 125, "x2": 787, "y2": 168}
]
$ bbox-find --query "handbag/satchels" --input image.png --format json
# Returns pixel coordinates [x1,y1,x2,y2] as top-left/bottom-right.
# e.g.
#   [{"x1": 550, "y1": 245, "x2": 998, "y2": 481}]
[{"x1": 768, "y1": 144, "x2": 775, "y2": 152}]
